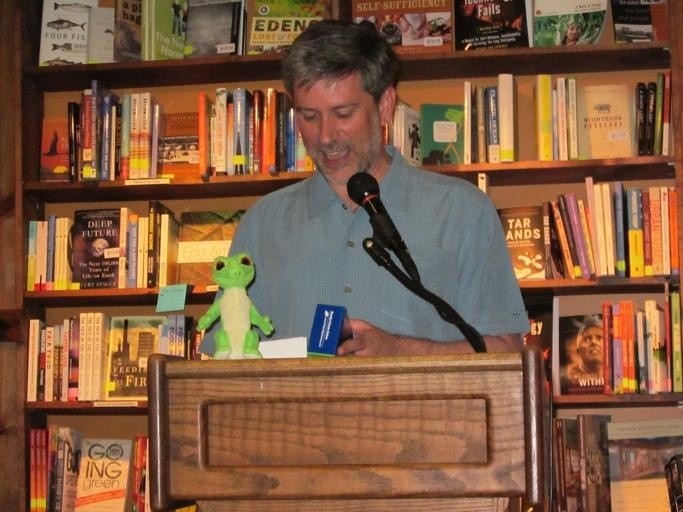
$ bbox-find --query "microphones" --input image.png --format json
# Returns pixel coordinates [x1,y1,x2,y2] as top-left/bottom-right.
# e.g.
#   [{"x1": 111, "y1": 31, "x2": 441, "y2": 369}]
[{"x1": 346, "y1": 172, "x2": 421, "y2": 282}]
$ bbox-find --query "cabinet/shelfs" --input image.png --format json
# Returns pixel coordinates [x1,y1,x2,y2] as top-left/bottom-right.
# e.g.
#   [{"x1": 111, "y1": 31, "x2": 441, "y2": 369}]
[{"x1": 13, "y1": 0, "x2": 682, "y2": 512}]
